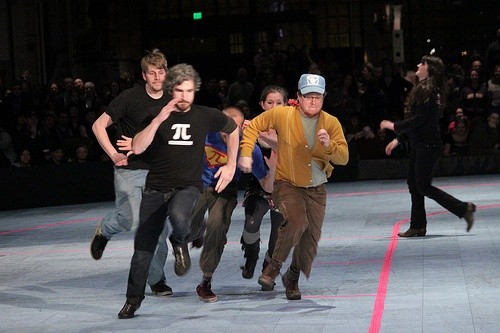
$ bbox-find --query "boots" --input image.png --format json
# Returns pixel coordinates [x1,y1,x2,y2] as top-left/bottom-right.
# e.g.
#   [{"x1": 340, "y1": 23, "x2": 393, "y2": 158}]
[
  {"x1": 262, "y1": 250, "x2": 276, "y2": 291},
  {"x1": 258, "y1": 261, "x2": 280, "y2": 290},
  {"x1": 241, "y1": 236, "x2": 260, "y2": 279},
  {"x1": 282, "y1": 267, "x2": 301, "y2": 300}
]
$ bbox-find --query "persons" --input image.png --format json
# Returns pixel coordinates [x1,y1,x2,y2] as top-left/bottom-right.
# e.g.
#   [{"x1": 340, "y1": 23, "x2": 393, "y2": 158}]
[
  {"x1": 0, "y1": 69, "x2": 147, "y2": 169},
  {"x1": 240, "y1": 84, "x2": 289, "y2": 286},
  {"x1": 380, "y1": 55, "x2": 477, "y2": 238},
  {"x1": 239, "y1": 74, "x2": 349, "y2": 300},
  {"x1": 438, "y1": 28, "x2": 500, "y2": 157},
  {"x1": 118, "y1": 63, "x2": 239, "y2": 318},
  {"x1": 192, "y1": 35, "x2": 419, "y2": 159},
  {"x1": 90, "y1": 49, "x2": 174, "y2": 296},
  {"x1": 189, "y1": 107, "x2": 278, "y2": 308}
]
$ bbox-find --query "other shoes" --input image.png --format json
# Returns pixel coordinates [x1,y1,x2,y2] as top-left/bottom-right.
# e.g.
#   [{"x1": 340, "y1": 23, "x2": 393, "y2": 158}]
[
  {"x1": 398, "y1": 227, "x2": 426, "y2": 237},
  {"x1": 464, "y1": 203, "x2": 476, "y2": 232}
]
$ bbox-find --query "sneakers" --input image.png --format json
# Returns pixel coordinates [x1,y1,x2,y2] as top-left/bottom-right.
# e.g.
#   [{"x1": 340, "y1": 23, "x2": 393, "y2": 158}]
[
  {"x1": 90, "y1": 219, "x2": 111, "y2": 260},
  {"x1": 118, "y1": 302, "x2": 141, "y2": 319},
  {"x1": 170, "y1": 238, "x2": 191, "y2": 276},
  {"x1": 151, "y1": 280, "x2": 172, "y2": 296},
  {"x1": 192, "y1": 237, "x2": 205, "y2": 248},
  {"x1": 196, "y1": 286, "x2": 217, "y2": 302}
]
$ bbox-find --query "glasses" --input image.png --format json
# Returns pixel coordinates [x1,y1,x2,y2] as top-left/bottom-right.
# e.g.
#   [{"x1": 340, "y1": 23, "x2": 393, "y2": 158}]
[{"x1": 303, "y1": 95, "x2": 322, "y2": 102}]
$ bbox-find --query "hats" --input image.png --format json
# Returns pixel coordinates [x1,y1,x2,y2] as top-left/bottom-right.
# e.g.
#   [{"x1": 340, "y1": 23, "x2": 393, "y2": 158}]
[{"x1": 298, "y1": 74, "x2": 325, "y2": 94}]
